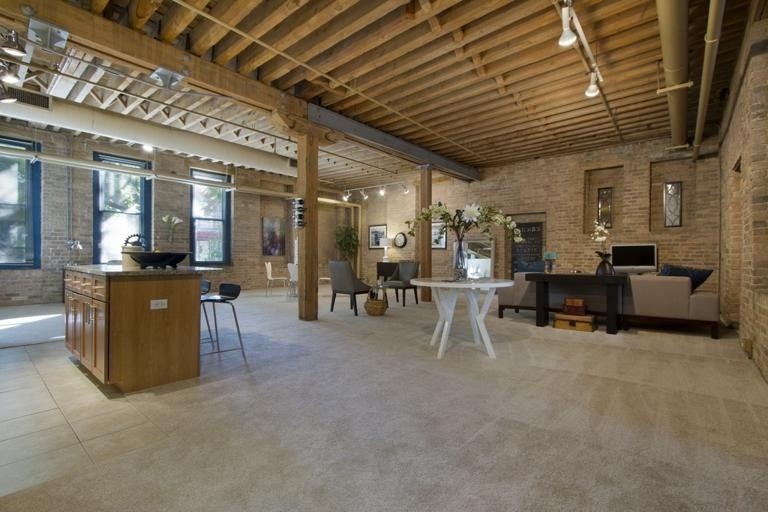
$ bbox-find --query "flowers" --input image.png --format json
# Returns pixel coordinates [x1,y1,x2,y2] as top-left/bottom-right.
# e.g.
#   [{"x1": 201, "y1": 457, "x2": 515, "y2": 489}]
[
  {"x1": 404, "y1": 201, "x2": 525, "y2": 242},
  {"x1": 161, "y1": 214, "x2": 183, "y2": 243},
  {"x1": 544, "y1": 252, "x2": 559, "y2": 259},
  {"x1": 590, "y1": 217, "x2": 610, "y2": 254}
]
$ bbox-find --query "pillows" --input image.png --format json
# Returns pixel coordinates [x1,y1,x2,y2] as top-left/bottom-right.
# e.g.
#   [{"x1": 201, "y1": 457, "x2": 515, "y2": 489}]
[
  {"x1": 657, "y1": 264, "x2": 713, "y2": 292},
  {"x1": 516, "y1": 262, "x2": 544, "y2": 272}
]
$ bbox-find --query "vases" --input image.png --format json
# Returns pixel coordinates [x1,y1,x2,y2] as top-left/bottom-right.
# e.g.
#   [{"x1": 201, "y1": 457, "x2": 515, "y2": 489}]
[
  {"x1": 545, "y1": 259, "x2": 556, "y2": 273},
  {"x1": 453, "y1": 241, "x2": 468, "y2": 281}
]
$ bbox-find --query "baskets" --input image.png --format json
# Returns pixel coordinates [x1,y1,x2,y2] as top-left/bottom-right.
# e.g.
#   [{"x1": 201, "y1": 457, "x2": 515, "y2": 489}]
[{"x1": 364, "y1": 286, "x2": 389, "y2": 316}]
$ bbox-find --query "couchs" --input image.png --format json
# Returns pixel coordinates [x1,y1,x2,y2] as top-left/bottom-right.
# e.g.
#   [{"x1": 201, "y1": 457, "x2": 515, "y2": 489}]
[
  {"x1": 622, "y1": 272, "x2": 720, "y2": 340},
  {"x1": 495, "y1": 272, "x2": 622, "y2": 330}
]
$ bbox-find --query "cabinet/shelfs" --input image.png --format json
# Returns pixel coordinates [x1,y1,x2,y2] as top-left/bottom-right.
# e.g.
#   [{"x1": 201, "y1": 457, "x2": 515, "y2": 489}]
[{"x1": 63, "y1": 264, "x2": 203, "y2": 399}]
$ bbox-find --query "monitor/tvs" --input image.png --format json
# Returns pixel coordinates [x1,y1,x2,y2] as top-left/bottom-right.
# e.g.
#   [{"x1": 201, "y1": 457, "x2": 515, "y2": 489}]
[{"x1": 611, "y1": 245, "x2": 658, "y2": 275}]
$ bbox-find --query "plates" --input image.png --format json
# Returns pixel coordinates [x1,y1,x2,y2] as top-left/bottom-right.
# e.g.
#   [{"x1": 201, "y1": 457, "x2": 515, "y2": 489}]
[{"x1": 121, "y1": 251, "x2": 192, "y2": 270}]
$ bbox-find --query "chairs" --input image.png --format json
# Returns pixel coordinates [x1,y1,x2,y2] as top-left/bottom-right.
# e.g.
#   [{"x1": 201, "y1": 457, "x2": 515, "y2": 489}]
[
  {"x1": 200, "y1": 283, "x2": 244, "y2": 355},
  {"x1": 287, "y1": 263, "x2": 298, "y2": 296},
  {"x1": 265, "y1": 262, "x2": 288, "y2": 297},
  {"x1": 385, "y1": 260, "x2": 419, "y2": 307},
  {"x1": 329, "y1": 260, "x2": 371, "y2": 316},
  {"x1": 200, "y1": 280, "x2": 213, "y2": 344}
]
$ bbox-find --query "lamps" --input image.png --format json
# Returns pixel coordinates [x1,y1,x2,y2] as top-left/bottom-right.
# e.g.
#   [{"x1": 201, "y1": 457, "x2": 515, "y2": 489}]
[
  {"x1": 585, "y1": 63, "x2": 600, "y2": 97},
  {"x1": 398, "y1": 182, "x2": 410, "y2": 195},
  {"x1": 0, "y1": 28, "x2": 27, "y2": 57},
  {"x1": 374, "y1": 185, "x2": 385, "y2": 198},
  {"x1": 0, "y1": 83, "x2": 17, "y2": 103},
  {"x1": 379, "y1": 238, "x2": 392, "y2": 262},
  {"x1": 0, "y1": 61, "x2": 20, "y2": 85},
  {"x1": 360, "y1": 188, "x2": 368, "y2": 201},
  {"x1": 558, "y1": 0, "x2": 577, "y2": 47},
  {"x1": 342, "y1": 191, "x2": 353, "y2": 202}
]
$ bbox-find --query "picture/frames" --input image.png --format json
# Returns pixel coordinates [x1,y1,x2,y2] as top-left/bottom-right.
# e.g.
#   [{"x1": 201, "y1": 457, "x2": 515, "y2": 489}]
[
  {"x1": 452, "y1": 238, "x2": 495, "y2": 293},
  {"x1": 597, "y1": 188, "x2": 613, "y2": 228},
  {"x1": 431, "y1": 222, "x2": 447, "y2": 249},
  {"x1": 368, "y1": 225, "x2": 387, "y2": 248},
  {"x1": 663, "y1": 182, "x2": 682, "y2": 227},
  {"x1": 393, "y1": 232, "x2": 407, "y2": 248},
  {"x1": 262, "y1": 217, "x2": 286, "y2": 256}
]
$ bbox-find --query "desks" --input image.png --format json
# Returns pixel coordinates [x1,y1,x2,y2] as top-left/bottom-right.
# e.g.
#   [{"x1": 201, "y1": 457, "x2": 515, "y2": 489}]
[
  {"x1": 377, "y1": 262, "x2": 398, "y2": 282},
  {"x1": 525, "y1": 273, "x2": 627, "y2": 334},
  {"x1": 410, "y1": 277, "x2": 516, "y2": 361}
]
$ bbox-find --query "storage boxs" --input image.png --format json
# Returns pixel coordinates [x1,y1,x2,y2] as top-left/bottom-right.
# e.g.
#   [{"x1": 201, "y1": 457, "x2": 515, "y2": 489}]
[
  {"x1": 563, "y1": 297, "x2": 586, "y2": 316},
  {"x1": 553, "y1": 312, "x2": 598, "y2": 332}
]
